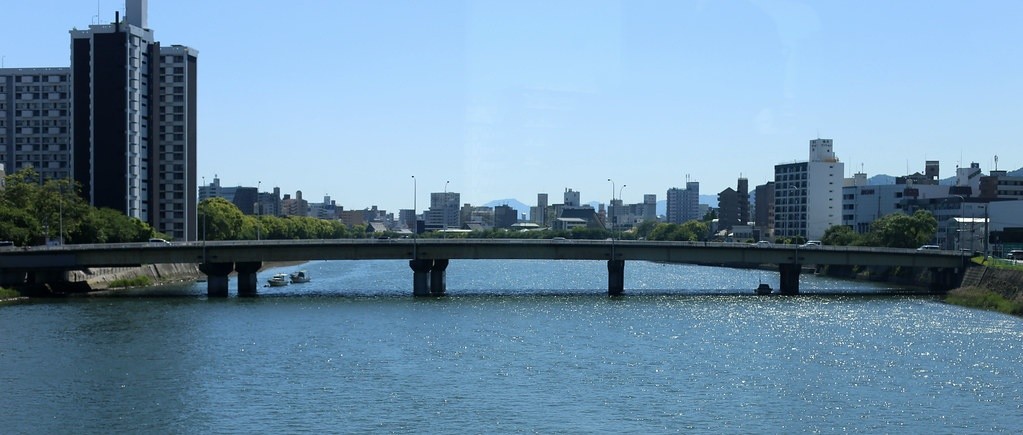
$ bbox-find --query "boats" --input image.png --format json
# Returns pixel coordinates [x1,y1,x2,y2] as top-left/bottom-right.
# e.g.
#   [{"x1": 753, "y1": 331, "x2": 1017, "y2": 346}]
[
  {"x1": 267, "y1": 273, "x2": 289, "y2": 286},
  {"x1": 755, "y1": 283, "x2": 773, "y2": 295},
  {"x1": 289, "y1": 270, "x2": 310, "y2": 283}
]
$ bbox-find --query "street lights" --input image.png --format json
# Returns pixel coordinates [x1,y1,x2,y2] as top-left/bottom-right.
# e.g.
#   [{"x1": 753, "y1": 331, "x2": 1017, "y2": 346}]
[
  {"x1": 619, "y1": 185, "x2": 626, "y2": 200},
  {"x1": 444, "y1": 181, "x2": 449, "y2": 237},
  {"x1": 607, "y1": 178, "x2": 614, "y2": 260},
  {"x1": 937, "y1": 199, "x2": 947, "y2": 246},
  {"x1": 58, "y1": 183, "x2": 62, "y2": 245},
  {"x1": 953, "y1": 195, "x2": 963, "y2": 272},
  {"x1": 412, "y1": 175, "x2": 416, "y2": 260},
  {"x1": 202, "y1": 177, "x2": 205, "y2": 264},
  {"x1": 258, "y1": 181, "x2": 261, "y2": 240},
  {"x1": 790, "y1": 185, "x2": 798, "y2": 263}
]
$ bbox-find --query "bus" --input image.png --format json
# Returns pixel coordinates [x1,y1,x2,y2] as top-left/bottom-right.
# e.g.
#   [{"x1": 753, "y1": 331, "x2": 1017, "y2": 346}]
[{"x1": 0, "y1": 241, "x2": 14, "y2": 252}]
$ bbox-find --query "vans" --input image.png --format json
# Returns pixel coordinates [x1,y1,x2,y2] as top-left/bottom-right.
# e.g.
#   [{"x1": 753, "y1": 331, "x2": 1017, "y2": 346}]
[
  {"x1": 149, "y1": 239, "x2": 171, "y2": 247},
  {"x1": 1007, "y1": 250, "x2": 1023, "y2": 260},
  {"x1": 916, "y1": 245, "x2": 939, "y2": 253},
  {"x1": 958, "y1": 249, "x2": 970, "y2": 253}
]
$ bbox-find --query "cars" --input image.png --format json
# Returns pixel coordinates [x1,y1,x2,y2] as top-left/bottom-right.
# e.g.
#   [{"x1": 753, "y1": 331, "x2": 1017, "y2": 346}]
[
  {"x1": 798, "y1": 241, "x2": 822, "y2": 249},
  {"x1": 378, "y1": 237, "x2": 397, "y2": 243},
  {"x1": 750, "y1": 241, "x2": 776, "y2": 248},
  {"x1": 553, "y1": 237, "x2": 573, "y2": 243}
]
